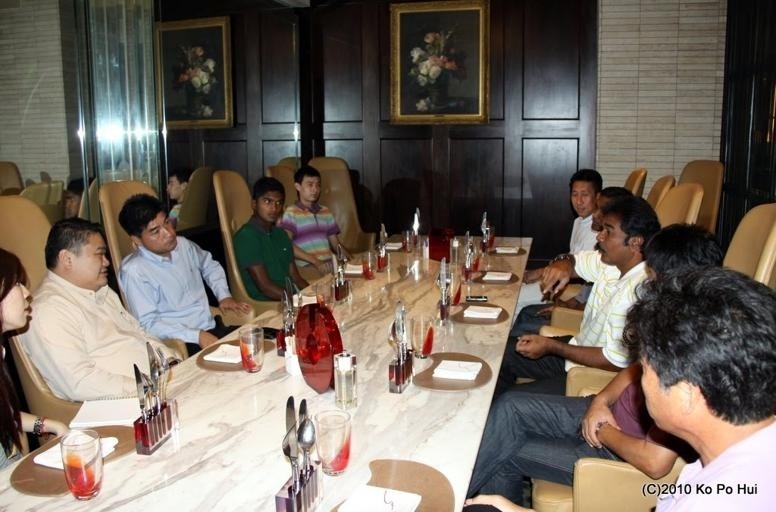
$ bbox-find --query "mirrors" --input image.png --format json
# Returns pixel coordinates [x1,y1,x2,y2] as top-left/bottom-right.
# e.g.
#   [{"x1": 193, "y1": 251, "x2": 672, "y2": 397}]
[{"x1": 0, "y1": 0, "x2": 218, "y2": 253}]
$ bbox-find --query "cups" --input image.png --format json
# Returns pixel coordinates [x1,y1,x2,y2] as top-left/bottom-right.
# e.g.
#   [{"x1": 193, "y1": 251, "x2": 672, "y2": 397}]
[
  {"x1": 132, "y1": 206, "x2": 497, "y2": 510},
  {"x1": 58, "y1": 428, "x2": 105, "y2": 501}
]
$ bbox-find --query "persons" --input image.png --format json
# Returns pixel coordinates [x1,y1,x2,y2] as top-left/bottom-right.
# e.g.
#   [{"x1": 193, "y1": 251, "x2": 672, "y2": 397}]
[
  {"x1": 233, "y1": 177, "x2": 310, "y2": 313},
  {"x1": 460, "y1": 220, "x2": 722, "y2": 508},
  {"x1": 460, "y1": 260, "x2": 773, "y2": 512},
  {"x1": 15, "y1": 219, "x2": 184, "y2": 403},
  {"x1": 0, "y1": 246, "x2": 71, "y2": 478},
  {"x1": 508, "y1": 185, "x2": 638, "y2": 340},
  {"x1": 62, "y1": 174, "x2": 94, "y2": 218},
  {"x1": 280, "y1": 166, "x2": 355, "y2": 287},
  {"x1": 116, "y1": 190, "x2": 252, "y2": 359},
  {"x1": 513, "y1": 168, "x2": 603, "y2": 323},
  {"x1": 486, "y1": 196, "x2": 660, "y2": 405},
  {"x1": 161, "y1": 166, "x2": 192, "y2": 231}
]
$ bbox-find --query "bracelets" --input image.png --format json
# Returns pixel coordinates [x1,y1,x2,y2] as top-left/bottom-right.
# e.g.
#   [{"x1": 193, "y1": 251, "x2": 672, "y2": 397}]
[
  {"x1": 551, "y1": 254, "x2": 575, "y2": 267},
  {"x1": 595, "y1": 421, "x2": 614, "y2": 435},
  {"x1": 33, "y1": 415, "x2": 49, "y2": 436}
]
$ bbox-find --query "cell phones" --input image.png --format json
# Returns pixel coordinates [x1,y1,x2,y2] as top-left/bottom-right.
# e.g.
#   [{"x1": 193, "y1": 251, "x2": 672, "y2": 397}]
[
  {"x1": 466, "y1": 296, "x2": 488, "y2": 302},
  {"x1": 255, "y1": 327, "x2": 281, "y2": 339}
]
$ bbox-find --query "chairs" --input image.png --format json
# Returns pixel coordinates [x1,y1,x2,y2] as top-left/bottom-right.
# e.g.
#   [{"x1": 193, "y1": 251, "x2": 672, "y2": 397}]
[
  {"x1": 0, "y1": 172, "x2": 776, "y2": 510},
  {"x1": 0, "y1": 155, "x2": 215, "y2": 231}
]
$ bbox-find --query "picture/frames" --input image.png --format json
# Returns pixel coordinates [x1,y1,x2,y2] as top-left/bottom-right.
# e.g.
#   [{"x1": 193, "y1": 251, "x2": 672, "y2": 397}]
[
  {"x1": 388, "y1": 3, "x2": 489, "y2": 126},
  {"x1": 152, "y1": 15, "x2": 234, "y2": 132}
]
